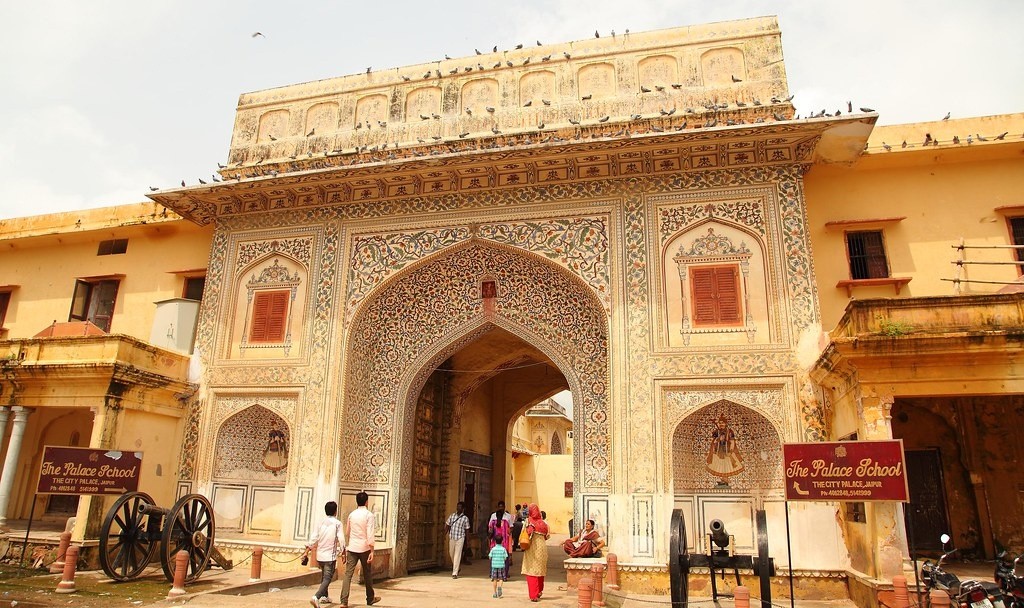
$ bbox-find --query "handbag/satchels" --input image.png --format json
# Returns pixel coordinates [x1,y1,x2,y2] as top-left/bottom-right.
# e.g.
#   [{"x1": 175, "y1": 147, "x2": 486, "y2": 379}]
[
  {"x1": 520, "y1": 518, "x2": 530, "y2": 550},
  {"x1": 592, "y1": 541, "x2": 602, "y2": 557},
  {"x1": 488, "y1": 520, "x2": 495, "y2": 541}
]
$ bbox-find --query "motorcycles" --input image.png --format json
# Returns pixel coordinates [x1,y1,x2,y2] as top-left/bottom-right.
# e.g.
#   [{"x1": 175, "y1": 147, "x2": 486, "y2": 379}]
[
  {"x1": 988, "y1": 538, "x2": 1024, "y2": 608},
  {"x1": 920, "y1": 534, "x2": 996, "y2": 608}
]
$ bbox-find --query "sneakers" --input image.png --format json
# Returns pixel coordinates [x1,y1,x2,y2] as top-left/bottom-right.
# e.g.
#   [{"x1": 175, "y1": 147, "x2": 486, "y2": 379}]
[
  {"x1": 310, "y1": 595, "x2": 320, "y2": 608},
  {"x1": 319, "y1": 596, "x2": 332, "y2": 602}
]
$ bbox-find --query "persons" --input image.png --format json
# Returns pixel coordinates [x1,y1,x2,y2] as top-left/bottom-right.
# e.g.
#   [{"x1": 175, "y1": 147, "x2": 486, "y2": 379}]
[
  {"x1": 518, "y1": 504, "x2": 550, "y2": 602},
  {"x1": 299, "y1": 501, "x2": 346, "y2": 608},
  {"x1": 444, "y1": 501, "x2": 471, "y2": 579},
  {"x1": 339, "y1": 492, "x2": 382, "y2": 607},
  {"x1": 560, "y1": 519, "x2": 605, "y2": 558},
  {"x1": 487, "y1": 501, "x2": 546, "y2": 582},
  {"x1": 488, "y1": 535, "x2": 509, "y2": 598}
]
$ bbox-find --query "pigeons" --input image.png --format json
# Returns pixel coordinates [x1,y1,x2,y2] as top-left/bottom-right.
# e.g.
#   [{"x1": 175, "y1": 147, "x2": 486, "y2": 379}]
[{"x1": 150, "y1": 27, "x2": 1009, "y2": 194}]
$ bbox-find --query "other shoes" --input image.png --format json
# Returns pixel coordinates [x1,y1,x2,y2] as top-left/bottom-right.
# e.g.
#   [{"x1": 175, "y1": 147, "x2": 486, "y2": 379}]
[
  {"x1": 452, "y1": 575, "x2": 457, "y2": 579},
  {"x1": 340, "y1": 604, "x2": 348, "y2": 608},
  {"x1": 498, "y1": 586, "x2": 502, "y2": 596},
  {"x1": 493, "y1": 594, "x2": 497, "y2": 597},
  {"x1": 537, "y1": 591, "x2": 542, "y2": 598},
  {"x1": 368, "y1": 597, "x2": 381, "y2": 605},
  {"x1": 531, "y1": 598, "x2": 537, "y2": 602}
]
